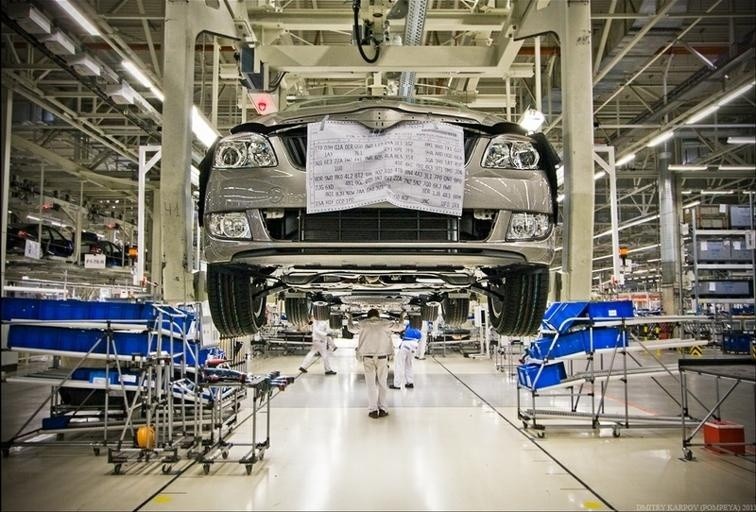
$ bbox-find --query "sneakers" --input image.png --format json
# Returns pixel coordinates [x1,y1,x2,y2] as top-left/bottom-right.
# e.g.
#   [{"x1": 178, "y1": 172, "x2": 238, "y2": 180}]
[
  {"x1": 299, "y1": 367, "x2": 308, "y2": 373},
  {"x1": 379, "y1": 409, "x2": 388, "y2": 416},
  {"x1": 390, "y1": 384, "x2": 400, "y2": 389},
  {"x1": 325, "y1": 370, "x2": 336, "y2": 374},
  {"x1": 405, "y1": 383, "x2": 413, "y2": 388},
  {"x1": 370, "y1": 411, "x2": 377, "y2": 417}
]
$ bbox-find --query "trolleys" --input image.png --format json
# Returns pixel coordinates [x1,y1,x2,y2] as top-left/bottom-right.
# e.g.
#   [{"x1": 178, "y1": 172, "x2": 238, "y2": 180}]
[
  {"x1": 514, "y1": 299, "x2": 722, "y2": 439},
  {"x1": 195, "y1": 369, "x2": 295, "y2": 475},
  {"x1": 104, "y1": 302, "x2": 191, "y2": 473},
  {"x1": 677, "y1": 357, "x2": 756, "y2": 460}
]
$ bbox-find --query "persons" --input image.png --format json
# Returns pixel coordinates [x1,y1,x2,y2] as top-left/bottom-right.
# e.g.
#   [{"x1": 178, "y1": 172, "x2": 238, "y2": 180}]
[
  {"x1": 302, "y1": 320, "x2": 337, "y2": 375},
  {"x1": 389, "y1": 326, "x2": 421, "y2": 390},
  {"x1": 344, "y1": 309, "x2": 407, "y2": 420},
  {"x1": 416, "y1": 321, "x2": 432, "y2": 361},
  {"x1": 327, "y1": 336, "x2": 339, "y2": 353}
]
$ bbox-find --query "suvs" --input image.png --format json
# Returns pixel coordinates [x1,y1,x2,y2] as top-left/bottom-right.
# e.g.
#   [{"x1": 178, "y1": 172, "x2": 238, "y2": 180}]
[{"x1": 197, "y1": 94, "x2": 560, "y2": 339}]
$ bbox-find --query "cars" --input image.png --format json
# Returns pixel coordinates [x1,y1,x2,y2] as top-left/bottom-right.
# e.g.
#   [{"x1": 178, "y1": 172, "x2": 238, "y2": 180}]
[
  {"x1": 6, "y1": 223, "x2": 74, "y2": 257},
  {"x1": 80, "y1": 240, "x2": 129, "y2": 266}
]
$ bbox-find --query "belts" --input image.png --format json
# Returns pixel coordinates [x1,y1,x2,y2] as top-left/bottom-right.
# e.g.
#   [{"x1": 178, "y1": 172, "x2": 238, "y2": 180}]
[{"x1": 363, "y1": 355, "x2": 386, "y2": 358}]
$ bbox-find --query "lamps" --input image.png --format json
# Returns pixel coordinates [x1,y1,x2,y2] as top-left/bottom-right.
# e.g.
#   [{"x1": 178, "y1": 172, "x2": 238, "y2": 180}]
[
  {"x1": 57, "y1": 0, "x2": 105, "y2": 40},
  {"x1": 615, "y1": 153, "x2": 639, "y2": 168},
  {"x1": 620, "y1": 245, "x2": 662, "y2": 257},
  {"x1": 646, "y1": 129, "x2": 673, "y2": 149},
  {"x1": 153, "y1": 86, "x2": 163, "y2": 105},
  {"x1": 683, "y1": 190, "x2": 691, "y2": 197},
  {"x1": 593, "y1": 170, "x2": 608, "y2": 181},
  {"x1": 723, "y1": 134, "x2": 752, "y2": 148},
  {"x1": 685, "y1": 105, "x2": 719, "y2": 124},
  {"x1": 516, "y1": 108, "x2": 548, "y2": 134},
  {"x1": 668, "y1": 162, "x2": 708, "y2": 174},
  {"x1": 702, "y1": 189, "x2": 735, "y2": 194},
  {"x1": 718, "y1": 82, "x2": 750, "y2": 108},
  {"x1": 187, "y1": 105, "x2": 219, "y2": 147},
  {"x1": 618, "y1": 215, "x2": 662, "y2": 234},
  {"x1": 121, "y1": 57, "x2": 152, "y2": 90},
  {"x1": 719, "y1": 164, "x2": 752, "y2": 172},
  {"x1": 595, "y1": 228, "x2": 612, "y2": 242}
]
